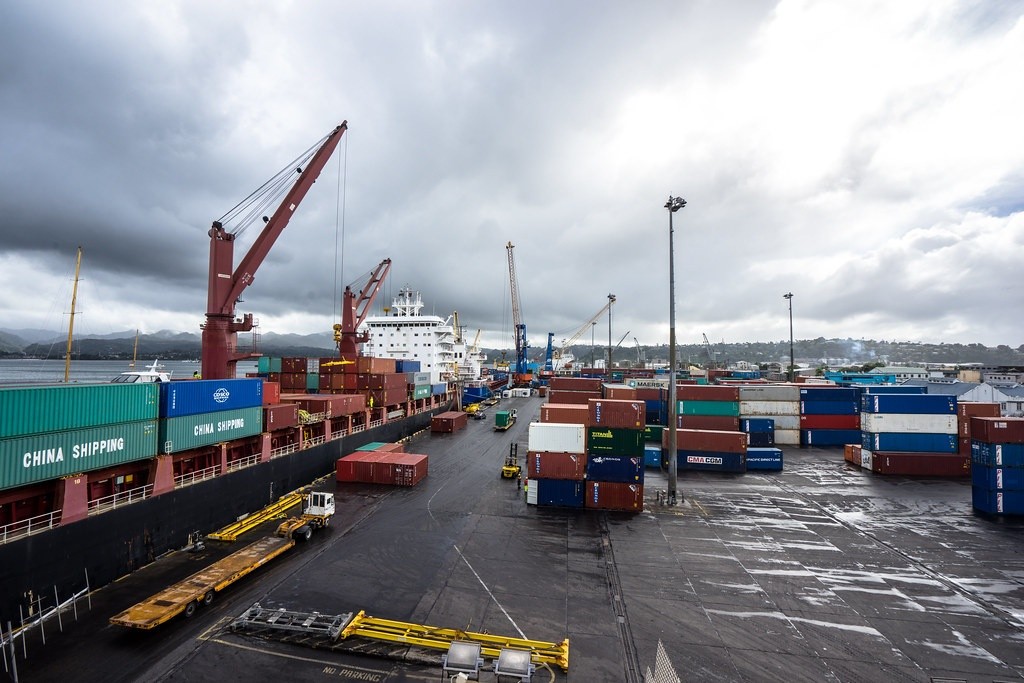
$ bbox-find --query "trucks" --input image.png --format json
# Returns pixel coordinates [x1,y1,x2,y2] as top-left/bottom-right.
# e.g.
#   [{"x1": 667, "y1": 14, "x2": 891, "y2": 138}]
[{"x1": 493, "y1": 408, "x2": 516, "y2": 430}]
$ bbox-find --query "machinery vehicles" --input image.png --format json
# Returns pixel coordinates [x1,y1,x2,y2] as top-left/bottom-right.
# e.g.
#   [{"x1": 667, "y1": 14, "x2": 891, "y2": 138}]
[
  {"x1": 538, "y1": 299, "x2": 616, "y2": 386},
  {"x1": 501, "y1": 443, "x2": 520, "y2": 478},
  {"x1": 497, "y1": 241, "x2": 534, "y2": 395}
]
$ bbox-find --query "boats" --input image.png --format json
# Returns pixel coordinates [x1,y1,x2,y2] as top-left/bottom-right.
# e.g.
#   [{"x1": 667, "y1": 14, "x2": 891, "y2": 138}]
[{"x1": 0, "y1": 285, "x2": 495, "y2": 649}]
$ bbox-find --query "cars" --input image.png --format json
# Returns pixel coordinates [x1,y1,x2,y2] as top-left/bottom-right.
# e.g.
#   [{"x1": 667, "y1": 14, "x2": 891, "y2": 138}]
[
  {"x1": 467, "y1": 404, "x2": 479, "y2": 412},
  {"x1": 475, "y1": 412, "x2": 485, "y2": 420}
]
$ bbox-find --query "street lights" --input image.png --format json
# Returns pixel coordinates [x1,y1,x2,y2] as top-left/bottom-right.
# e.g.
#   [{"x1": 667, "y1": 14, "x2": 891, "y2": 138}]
[
  {"x1": 784, "y1": 293, "x2": 794, "y2": 368},
  {"x1": 666, "y1": 195, "x2": 684, "y2": 506},
  {"x1": 607, "y1": 294, "x2": 616, "y2": 383}
]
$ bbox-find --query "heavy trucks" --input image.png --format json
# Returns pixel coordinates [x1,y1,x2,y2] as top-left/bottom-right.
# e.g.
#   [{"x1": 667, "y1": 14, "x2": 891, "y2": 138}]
[{"x1": 108, "y1": 493, "x2": 335, "y2": 628}]
